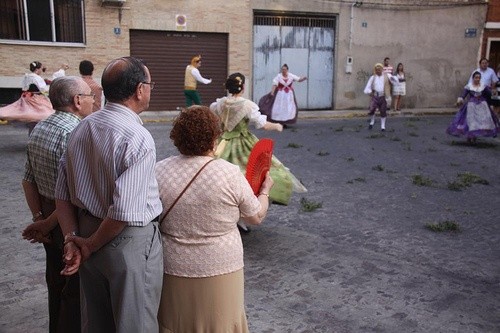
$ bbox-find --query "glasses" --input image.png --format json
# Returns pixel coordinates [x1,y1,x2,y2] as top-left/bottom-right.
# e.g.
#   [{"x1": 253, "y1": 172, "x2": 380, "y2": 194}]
[
  {"x1": 135, "y1": 82, "x2": 155, "y2": 90},
  {"x1": 71, "y1": 92, "x2": 97, "y2": 103}
]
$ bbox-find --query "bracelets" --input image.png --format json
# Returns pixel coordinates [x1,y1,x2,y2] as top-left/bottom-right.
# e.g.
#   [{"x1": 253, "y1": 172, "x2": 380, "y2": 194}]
[
  {"x1": 257, "y1": 192, "x2": 271, "y2": 198},
  {"x1": 64, "y1": 231, "x2": 79, "y2": 241},
  {"x1": 32, "y1": 211, "x2": 43, "y2": 222}
]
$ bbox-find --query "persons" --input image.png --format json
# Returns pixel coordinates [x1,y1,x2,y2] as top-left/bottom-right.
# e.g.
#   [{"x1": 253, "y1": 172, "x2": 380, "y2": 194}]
[
  {"x1": 364, "y1": 62, "x2": 399, "y2": 132},
  {"x1": 154, "y1": 105, "x2": 273, "y2": 333},
  {"x1": 446, "y1": 71, "x2": 500, "y2": 144},
  {"x1": 209, "y1": 72, "x2": 305, "y2": 233},
  {"x1": 392, "y1": 63, "x2": 406, "y2": 112},
  {"x1": 21, "y1": 76, "x2": 95, "y2": 333},
  {"x1": 382, "y1": 57, "x2": 393, "y2": 75},
  {"x1": 183, "y1": 56, "x2": 212, "y2": 107},
  {"x1": 54, "y1": 56, "x2": 163, "y2": 333},
  {"x1": 0, "y1": 60, "x2": 102, "y2": 136},
  {"x1": 468, "y1": 58, "x2": 499, "y2": 90},
  {"x1": 258, "y1": 64, "x2": 307, "y2": 128}
]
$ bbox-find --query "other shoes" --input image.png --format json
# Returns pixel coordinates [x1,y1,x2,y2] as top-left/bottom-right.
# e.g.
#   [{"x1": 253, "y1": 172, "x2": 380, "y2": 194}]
[
  {"x1": 238, "y1": 223, "x2": 250, "y2": 232},
  {"x1": 369, "y1": 124, "x2": 372, "y2": 129},
  {"x1": 381, "y1": 129, "x2": 385, "y2": 133}
]
